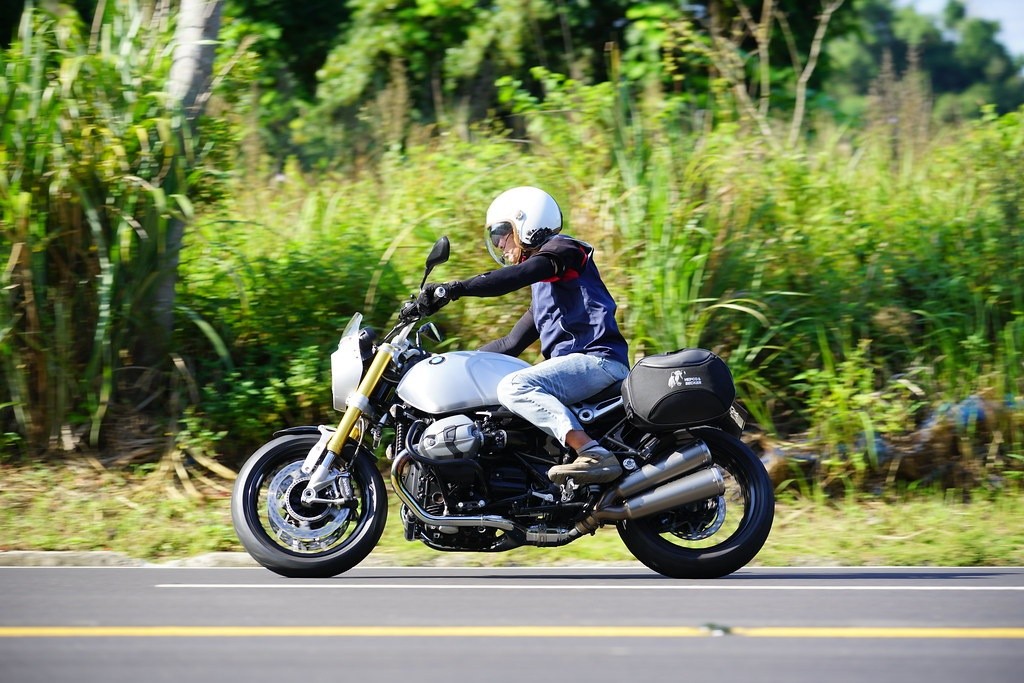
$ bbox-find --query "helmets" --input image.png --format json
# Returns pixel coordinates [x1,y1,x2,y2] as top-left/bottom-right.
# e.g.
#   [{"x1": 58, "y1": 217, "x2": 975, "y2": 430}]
[{"x1": 486, "y1": 186, "x2": 564, "y2": 250}]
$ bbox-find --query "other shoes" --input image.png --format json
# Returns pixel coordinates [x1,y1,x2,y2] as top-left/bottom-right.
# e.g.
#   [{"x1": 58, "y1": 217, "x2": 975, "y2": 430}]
[{"x1": 548, "y1": 451, "x2": 622, "y2": 483}]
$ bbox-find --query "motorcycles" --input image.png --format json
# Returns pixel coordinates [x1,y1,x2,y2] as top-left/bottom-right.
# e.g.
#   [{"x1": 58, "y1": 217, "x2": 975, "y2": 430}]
[{"x1": 229, "y1": 234, "x2": 775, "y2": 579}]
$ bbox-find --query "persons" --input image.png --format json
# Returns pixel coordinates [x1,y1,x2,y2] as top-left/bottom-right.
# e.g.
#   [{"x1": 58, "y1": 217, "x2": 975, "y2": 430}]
[{"x1": 418, "y1": 185, "x2": 631, "y2": 486}]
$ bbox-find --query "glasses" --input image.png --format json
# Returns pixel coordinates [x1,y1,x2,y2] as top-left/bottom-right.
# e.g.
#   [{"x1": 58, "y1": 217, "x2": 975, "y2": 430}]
[{"x1": 491, "y1": 224, "x2": 512, "y2": 248}]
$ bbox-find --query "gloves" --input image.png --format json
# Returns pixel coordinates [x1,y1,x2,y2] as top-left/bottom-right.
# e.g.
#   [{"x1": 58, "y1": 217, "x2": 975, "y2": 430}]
[{"x1": 417, "y1": 280, "x2": 463, "y2": 316}]
